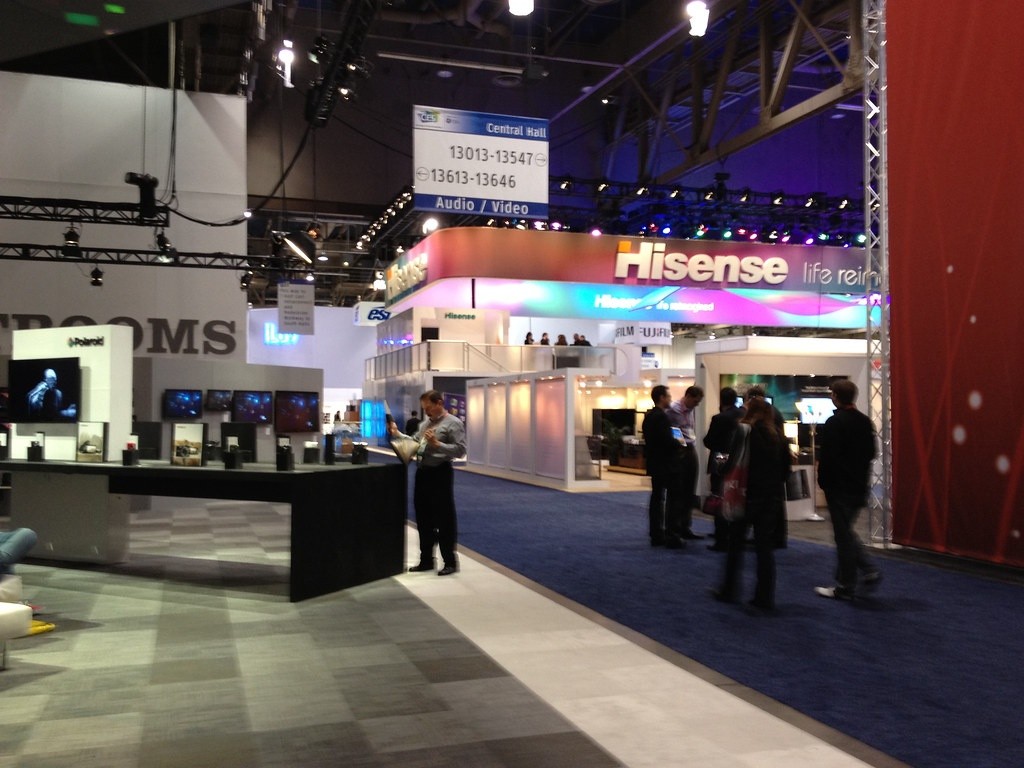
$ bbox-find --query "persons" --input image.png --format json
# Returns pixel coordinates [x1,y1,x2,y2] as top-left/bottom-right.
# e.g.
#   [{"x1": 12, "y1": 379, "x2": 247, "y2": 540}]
[
  {"x1": 44, "y1": 369, "x2": 62, "y2": 412},
  {"x1": 541, "y1": 333, "x2": 549, "y2": 346},
  {"x1": 525, "y1": 332, "x2": 534, "y2": 345},
  {"x1": 406, "y1": 410, "x2": 421, "y2": 436},
  {"x1": 335, "y1": 411, "x2": 340, "y2": 424},
  {"x1": 556, "y1": 335, "x2": 567, "y2": 346},
  {"x1": 813, "y1": 381, "x2": 879, "y2": 607},
  {"x1": 642, "y1": 386, "x2": 792, "y2": 615},
  {"x1": 574, "y1": 334, "x2": 582, "y2": 346},
  {"x1": 580, "y1": 335, "x2": 592, "y2": 347},
  {"x1": 391, "y1": 390, "x2": 466, "y2": 575}
]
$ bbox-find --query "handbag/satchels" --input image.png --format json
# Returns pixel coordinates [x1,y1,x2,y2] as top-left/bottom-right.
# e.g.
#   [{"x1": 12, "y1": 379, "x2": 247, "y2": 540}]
[
  {"x1": 390, "y1": 439, "x2": 421, "y2": 467},
  {"x1": 720, "y1": 480, "x2": 748, "y2": 523}
]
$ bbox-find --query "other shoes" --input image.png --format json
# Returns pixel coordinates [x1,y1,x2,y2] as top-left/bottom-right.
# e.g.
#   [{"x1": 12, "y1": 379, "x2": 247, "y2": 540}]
[
  {"x1": 682, "y1": 530, "x2": 704, "y2": 541},
  {"x1": 744, "y1": 600, "x2": 774, "y2": 612},
  {"x1": 712, "y1": 590, "x2": 735, "y2": 602},
  {"x1": 651, "y1": 535, "x2": 688, "y2": 548},
  {"x1": 817, "y1": 586, "x2": 853, "y2": 601},
  {"x1": 858, "y1": 570, "x2": 883, "y2": 602},
  {"x1": 706, "y1": 543, "x2": 724, "y2": 552},
  {"x1": 707, "y1": 532, "x2": 720, "y2": 539},
  {"x1": 409, "y1": 560, "x2": 436, "y2": 573},
  {"x1": 438, "y1": 563, "x2": 458, "y2": 575}
]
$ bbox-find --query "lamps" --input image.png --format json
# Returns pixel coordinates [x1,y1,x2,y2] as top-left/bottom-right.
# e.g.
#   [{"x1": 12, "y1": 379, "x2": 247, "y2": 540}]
[
  {"x1": 308, "y1": 34, "x2": 331, "y2": 64},
  {"x1": 487, "y1": 219, "x2": 571, "y2": 230},
  {"x1": 560, "y1": 179, "x2": 855, "y2": 213},
  {"x1": 157, "y1": 232, "x2": 172, "y2": 254},
  {"x1": 91, "y1": 268, "x2": 104, "y2": 287},
  {"x1": 62, "y1": 225, "x2": 82, "y2": 257},
  {"x1": 282, "y1": 228, "x2": 321, "y2": 265},
  {"x1": 240, "y1": 272, "x2": 255, "y2": 288},
  {"x1": 639, "y1": 222, "x2": 866, "y2": 247},
  {"x1": 317, "y1": 253, "x2": 329, "y2": 261},
  {"x1": 356, "y1": 189, "x2": 413, "y2": 250},
  {"x1": 435, "y1": 64, "x2": 454, "y2": 78}
]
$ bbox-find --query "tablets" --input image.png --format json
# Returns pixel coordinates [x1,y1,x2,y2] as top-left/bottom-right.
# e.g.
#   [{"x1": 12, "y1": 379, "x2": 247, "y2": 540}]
[{"x1": 671, "y1": 427, "x2": 687, "y2": 447}]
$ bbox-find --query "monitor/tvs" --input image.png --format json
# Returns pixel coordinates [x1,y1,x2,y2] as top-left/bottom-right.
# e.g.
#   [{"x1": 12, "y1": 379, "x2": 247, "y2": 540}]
[
  {"x1": 8, "y1": 356, "x2": 81, "y2": 423},
  {"x1": 163, "y1": 389, "x2": 321, "y2": 432},
  {"x1": 735, "y1": 397, "x2": 773, "y2": 407},
  {"x1": 801, "y1": 398, "x2": 837, "y2": 424}
]
